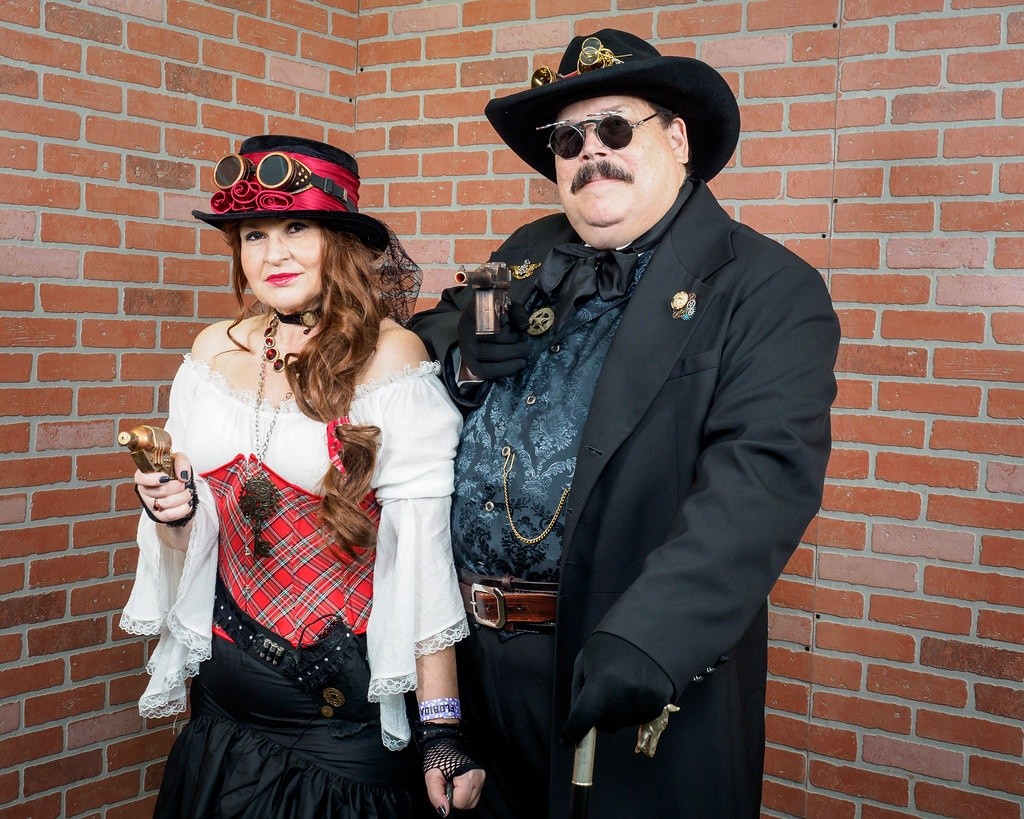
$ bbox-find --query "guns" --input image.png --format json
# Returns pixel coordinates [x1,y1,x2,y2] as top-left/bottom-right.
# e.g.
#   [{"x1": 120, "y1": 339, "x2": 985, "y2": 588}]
[
  {"x1": 454, "y1": 260, "x2": 518, "y2": 336},
  {"x1": 118, "y1": 424, "x2": 176, "y2": 480}
]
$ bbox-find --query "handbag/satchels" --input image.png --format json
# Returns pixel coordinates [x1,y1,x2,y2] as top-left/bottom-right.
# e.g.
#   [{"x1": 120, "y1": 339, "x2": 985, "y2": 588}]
[{"x1": 284, "y1": 621, "x2": 383, "y2": 722}]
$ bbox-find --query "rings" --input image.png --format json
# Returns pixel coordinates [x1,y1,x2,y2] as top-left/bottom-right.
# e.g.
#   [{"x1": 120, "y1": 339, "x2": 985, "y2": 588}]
[{"x1": 153, "y1": 497, "x2": 166, "y2": 512}]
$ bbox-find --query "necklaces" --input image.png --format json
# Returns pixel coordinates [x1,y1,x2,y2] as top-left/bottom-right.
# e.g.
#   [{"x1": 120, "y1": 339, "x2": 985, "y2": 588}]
[{"x1": 237, "y1": 304, "x2": 324, "y2": 557}]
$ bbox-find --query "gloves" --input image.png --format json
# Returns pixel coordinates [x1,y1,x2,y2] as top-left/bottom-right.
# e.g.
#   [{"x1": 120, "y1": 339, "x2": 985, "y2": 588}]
[
  {"x1": 458, "y1": 298, "x2": 529, "y2": 380},
  {"x1": 557, "y1": 632, "x2": 674, "y2": 749}
]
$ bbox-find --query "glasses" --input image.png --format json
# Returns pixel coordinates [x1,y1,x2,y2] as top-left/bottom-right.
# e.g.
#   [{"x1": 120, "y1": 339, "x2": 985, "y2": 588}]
[
  {"x1": 529, "y1": 37, "x2": 615, "y2": 88},
  {"x1": 536, "y1": 108, "x2": 661, "y2": 159},
  {"x1": 212, "y1": 153, "x2": 313, "y2": 195}
]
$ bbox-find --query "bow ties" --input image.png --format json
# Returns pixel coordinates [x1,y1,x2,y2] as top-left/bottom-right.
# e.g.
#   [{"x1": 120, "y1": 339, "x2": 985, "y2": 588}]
[{"x1": 539, "y1": 178, "x2": 694, "y2": 335}]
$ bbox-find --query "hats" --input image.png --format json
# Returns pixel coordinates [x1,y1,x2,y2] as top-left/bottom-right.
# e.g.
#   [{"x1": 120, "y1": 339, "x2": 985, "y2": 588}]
[
  {"x1": 483, "y1": 28, "x2": 741, "y2": 184},
  {"x1": 191, "y1": 135, "x2": 424, "y2": 326}
]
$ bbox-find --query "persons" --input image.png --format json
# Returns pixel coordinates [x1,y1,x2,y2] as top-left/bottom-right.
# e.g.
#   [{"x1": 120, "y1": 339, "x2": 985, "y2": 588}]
[
  {"x1": 119, "y1": 135, "x2": 486, "y2": 819},
  {"x1": 405, "y1": 29, "x2": 841, "y2": 819}
]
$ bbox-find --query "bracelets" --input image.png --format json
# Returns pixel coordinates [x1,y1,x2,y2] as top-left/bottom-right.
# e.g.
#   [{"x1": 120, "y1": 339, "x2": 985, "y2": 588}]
[{"x1": 418, "y1": 697, "x2": 461, "y2": 723}]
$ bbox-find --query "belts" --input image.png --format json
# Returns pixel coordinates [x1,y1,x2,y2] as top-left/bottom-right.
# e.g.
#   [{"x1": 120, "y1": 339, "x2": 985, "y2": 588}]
[{"x1": 457, "y1": 582, "x2": 558, "y2": 628}]
